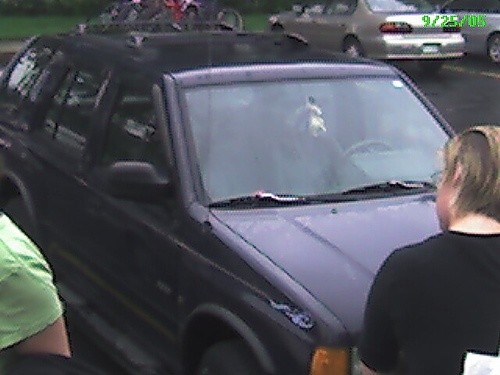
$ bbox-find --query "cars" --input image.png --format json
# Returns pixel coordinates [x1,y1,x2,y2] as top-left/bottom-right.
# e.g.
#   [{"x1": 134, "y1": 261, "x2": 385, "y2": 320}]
[
  {"x1": 434, "y1": 0, "x2": 500, "y2": 66},
  {"x1": 266, "y1": 0, "x2": 468, "y2": 64}
]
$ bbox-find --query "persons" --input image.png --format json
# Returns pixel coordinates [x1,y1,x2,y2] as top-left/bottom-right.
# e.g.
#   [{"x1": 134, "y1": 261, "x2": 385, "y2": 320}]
[
  {"x1": 358, "y1": 125, "x2": 500, "y2": 375},
  {"x1": 106, "y1": 0, "x2": 206, "y2": 34},
  {"x1": 0, "y1": 211, "x2": 71, "y2": 375}
]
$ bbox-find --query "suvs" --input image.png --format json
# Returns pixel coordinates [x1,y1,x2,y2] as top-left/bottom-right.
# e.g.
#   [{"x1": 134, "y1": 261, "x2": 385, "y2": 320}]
[{"x1": 0, "y1": 18, "x2": 464, "y2": 375}]
